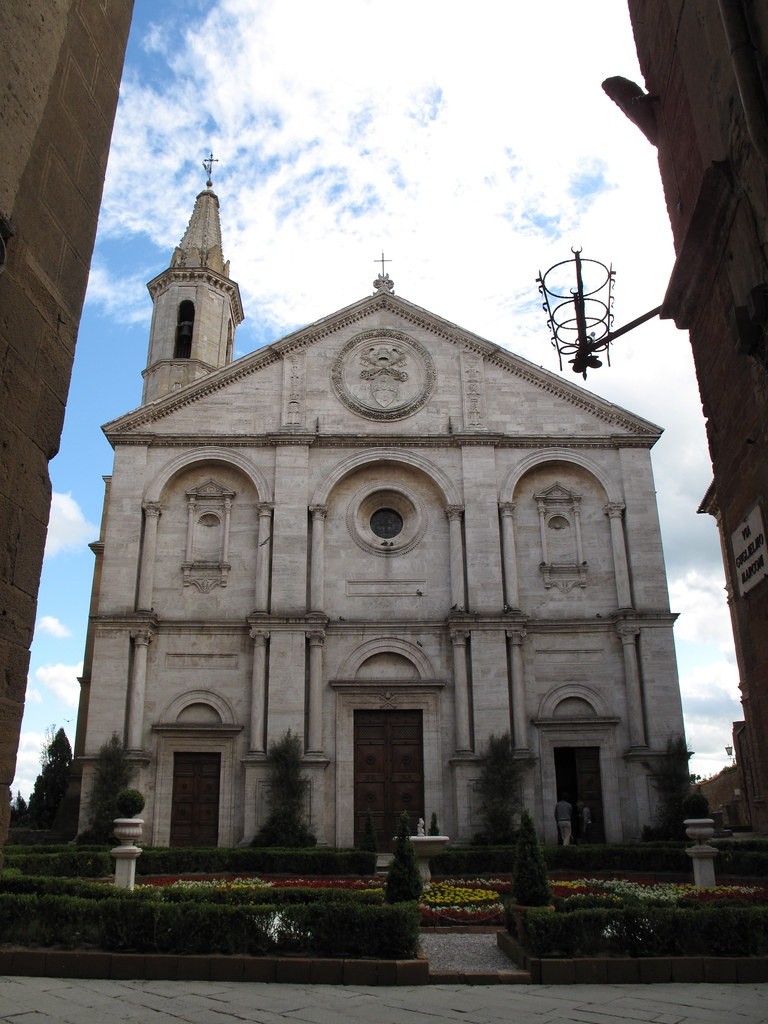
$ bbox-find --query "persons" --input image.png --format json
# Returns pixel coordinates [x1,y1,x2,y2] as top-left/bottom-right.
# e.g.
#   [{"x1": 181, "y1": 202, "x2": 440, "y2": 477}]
[
  {"x1": 554, "y1": 791, "x2": 572, "y2": 846},
  {"x1": 576, "y1": 800, "x2": 593, "y2": 846}
]
